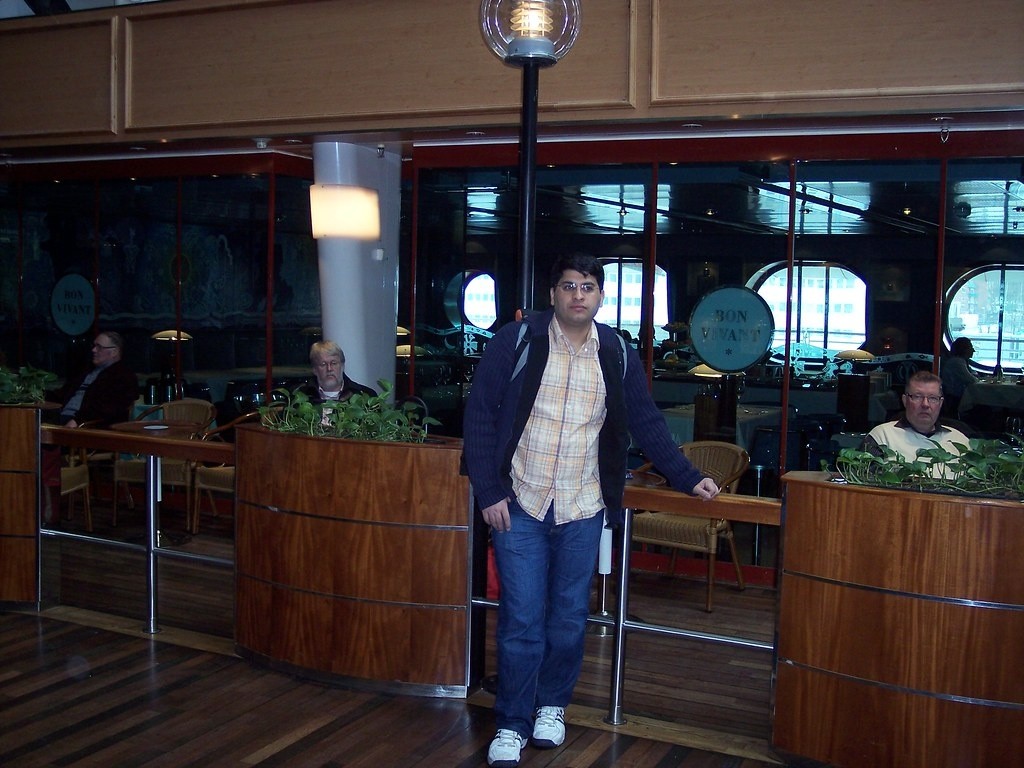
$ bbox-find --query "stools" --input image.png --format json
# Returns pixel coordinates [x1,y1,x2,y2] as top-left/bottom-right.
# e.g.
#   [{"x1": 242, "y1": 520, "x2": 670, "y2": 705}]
[{"x1": 748, "y1": 412, "x2": 847, "y2": 565}]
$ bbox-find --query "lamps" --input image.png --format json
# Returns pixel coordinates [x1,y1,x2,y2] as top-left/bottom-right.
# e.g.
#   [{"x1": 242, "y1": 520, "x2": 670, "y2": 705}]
[
  {"x1": 835, "y1": 346, "x2": 878, "y2": 372},
  {"x1": 690, "y1": 364, "x2": 744, "y2": 400},
  {"x1": 395, "y1": 346, "x2": 428, "y2": 375},
  {"x1": 153, "y1": 328, "x2": 192, "y2": 391},
  {"x1": 484, "y1": 0, "x2": 582, "y2": 317}
]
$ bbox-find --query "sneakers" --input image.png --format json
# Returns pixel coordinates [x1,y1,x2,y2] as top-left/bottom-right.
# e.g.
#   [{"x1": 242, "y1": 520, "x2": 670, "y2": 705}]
[
  {"x1": 488, "y1": 728, "x2": 528, "y2": 767},
  {"x1": 532, "y1": 706, "x2": 566, "y2": 747}
]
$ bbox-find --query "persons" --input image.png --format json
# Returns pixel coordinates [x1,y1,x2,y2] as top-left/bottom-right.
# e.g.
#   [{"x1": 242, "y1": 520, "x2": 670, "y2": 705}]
[
  {"x1": 41, "y1": 332, "x2": 140, "y2": 457},
  {"x1": 289, "y1": 340, "x2": 380, "y2": 428},
  {"x1": 459, "y1": 253, "x2": 721, "y2": 768},
  {"x1": 859, "y1": 371, "x2": 972, "y2": 480},
  {"x1": 941, "y1": 337, "x2": 980, "y2": 419}
]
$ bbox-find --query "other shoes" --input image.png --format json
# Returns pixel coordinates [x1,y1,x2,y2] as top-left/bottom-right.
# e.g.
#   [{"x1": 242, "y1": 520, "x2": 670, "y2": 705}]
[{"x1": 41, "y1": 522, "x2": 57, "y2": 537}]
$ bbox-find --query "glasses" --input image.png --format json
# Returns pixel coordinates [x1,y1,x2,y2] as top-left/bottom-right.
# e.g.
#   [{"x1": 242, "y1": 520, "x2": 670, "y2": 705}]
[
  {"x1": 555, "y1": 281, "x2": 599, "y2": 294},
  {"x1": 320, "y1": 360, "x2": 338, "y2": 367},
  {"x1": 90, "y1": 342, "x2": 116, "y2": 351},
  {"x1": 905, "y1": 393, "x2": 941, "y2": 403}
]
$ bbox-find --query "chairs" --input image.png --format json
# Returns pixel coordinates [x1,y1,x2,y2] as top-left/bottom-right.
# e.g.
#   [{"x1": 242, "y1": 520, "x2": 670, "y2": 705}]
[
  {"x1": 44, "y1": 407, "x2": 124, "y2": 532},
  {"x1": 190, "y1": 404, "x2": 298, "y2": 538},
  {"x1": 626, "y1": 441, "x2": 752, "y2": 613},
  {"x1": 105, "y1": 399, "x2": 218, "y2": 533}
]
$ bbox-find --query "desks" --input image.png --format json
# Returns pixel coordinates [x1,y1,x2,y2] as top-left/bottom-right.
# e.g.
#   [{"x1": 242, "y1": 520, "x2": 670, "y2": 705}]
[
  {"x1": 629, "y1": 404, "x2": 800, "y2": 473},
  {"x1": 129, "y1": 395, "x2": 219, "y2": 491},
  {"x1": 958, "y1": 375, "x2": 1024, "y2": 432}
]
